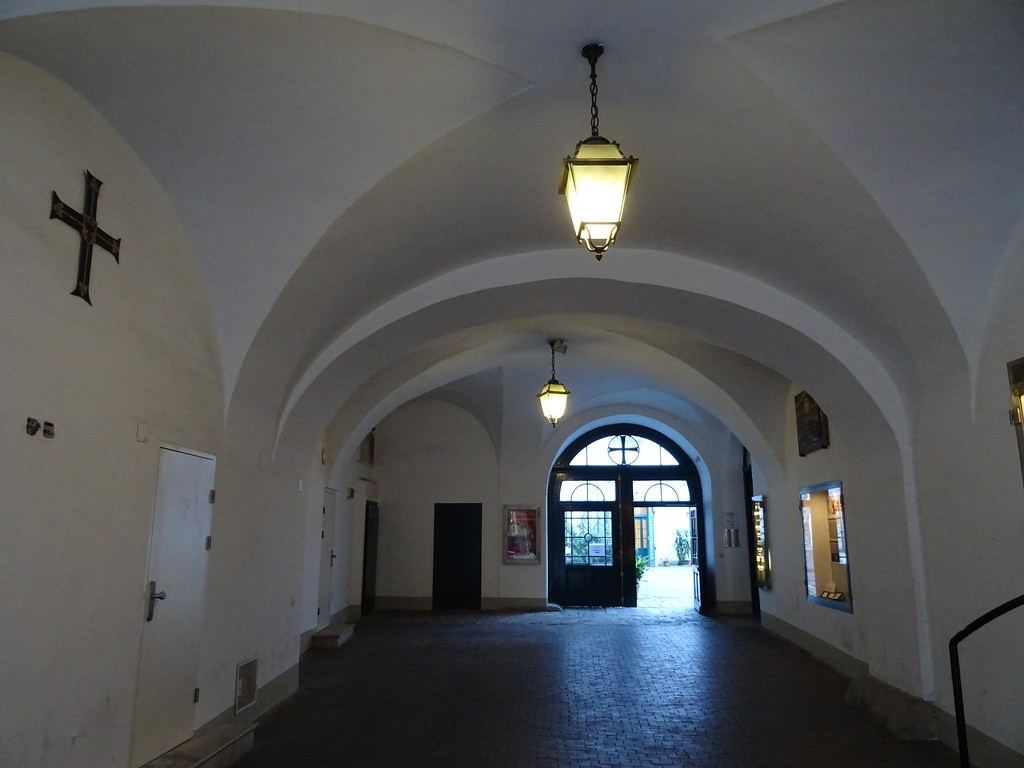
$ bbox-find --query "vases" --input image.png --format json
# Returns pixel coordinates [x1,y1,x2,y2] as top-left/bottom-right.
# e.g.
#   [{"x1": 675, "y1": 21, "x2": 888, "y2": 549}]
[{"x1": 663, "y1": 560, "x2": 670, "y2": 567}]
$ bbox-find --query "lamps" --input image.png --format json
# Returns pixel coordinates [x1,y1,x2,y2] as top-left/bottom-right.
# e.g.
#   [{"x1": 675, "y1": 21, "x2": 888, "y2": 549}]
[
  {"x1": 557, "y1": 41, "x2": 641, "y2": 262},
  {"x1": 536, "y1": 338, "x2": 571, "y2": 428}
]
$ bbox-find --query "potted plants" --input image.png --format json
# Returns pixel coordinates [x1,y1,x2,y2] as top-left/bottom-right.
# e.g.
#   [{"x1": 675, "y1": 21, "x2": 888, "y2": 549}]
[
  {"x1": 673, "y1": 529, "x2": 691, "y2": 565},
  {"x1": 635, "y1": 554, "x2": 650, "y2": 599}
]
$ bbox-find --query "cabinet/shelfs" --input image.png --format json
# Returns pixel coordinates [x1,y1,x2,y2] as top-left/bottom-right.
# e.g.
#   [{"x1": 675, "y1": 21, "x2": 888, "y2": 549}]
[{"x1": 828, "y1": 514, "x2": 846, "y2": 564}]
